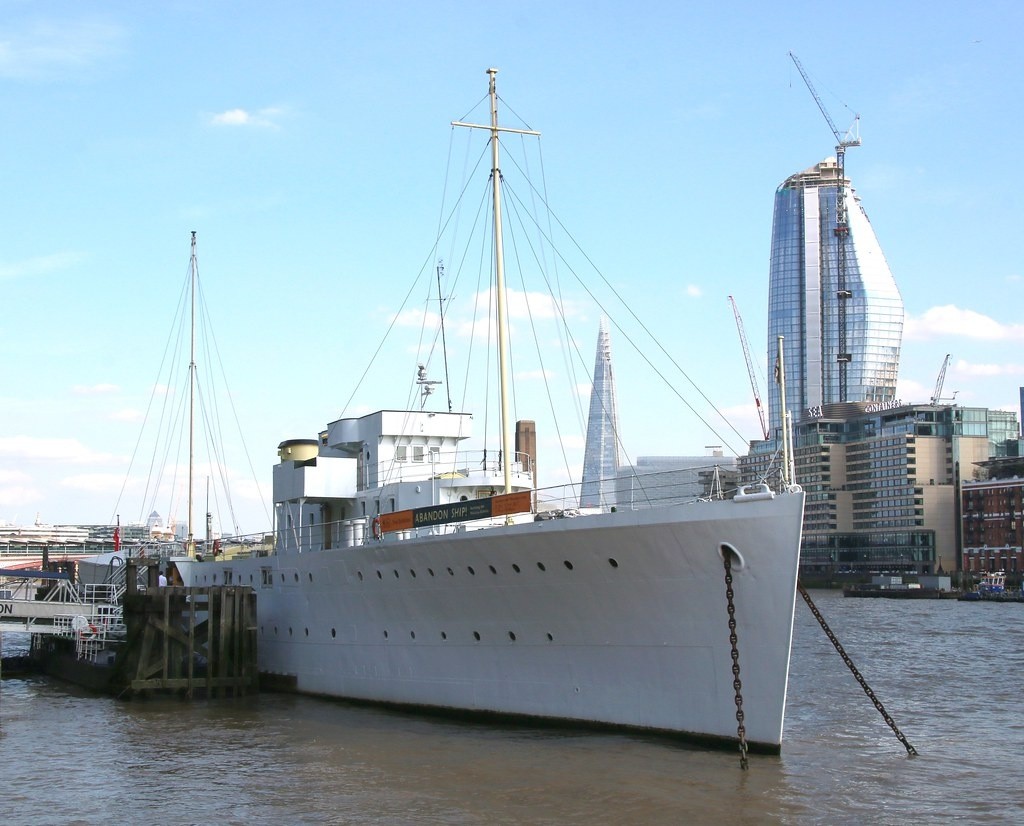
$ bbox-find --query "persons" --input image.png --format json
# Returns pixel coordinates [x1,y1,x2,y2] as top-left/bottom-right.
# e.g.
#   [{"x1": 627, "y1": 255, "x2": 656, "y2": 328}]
[{"x1": 158, "y1": 570, "x2": 167, "y2": 587}]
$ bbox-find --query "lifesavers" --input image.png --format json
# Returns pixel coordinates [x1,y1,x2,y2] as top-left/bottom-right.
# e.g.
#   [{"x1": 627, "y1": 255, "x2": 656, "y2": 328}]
[
  {"x1": 80, "y1": 624, "x2": 97, "y2": 640},
  {"x1": 212, "y1": 540, "x2": 220, "y2": 556},
  {"x1": 372, "y1": 517, "x2": 382, "y2": 537},
  {"x1": 184, "y1": 539, "x2": 196, "y2": 551}
]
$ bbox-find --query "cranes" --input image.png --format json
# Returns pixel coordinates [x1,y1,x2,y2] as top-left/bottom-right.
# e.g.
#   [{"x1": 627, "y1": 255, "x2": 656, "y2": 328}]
[
  {"x1": 929, "y1": 353, "x2": 958, "y2": 405},
  {"x1": 777, "y1": 30, "x2": 864, "y2": 398}
]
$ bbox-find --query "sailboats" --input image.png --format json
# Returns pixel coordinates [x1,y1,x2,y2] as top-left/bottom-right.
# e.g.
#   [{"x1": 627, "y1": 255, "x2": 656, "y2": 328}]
[{"x1": 75, "y1": 61, "x2": 811, "y2": 759}]
